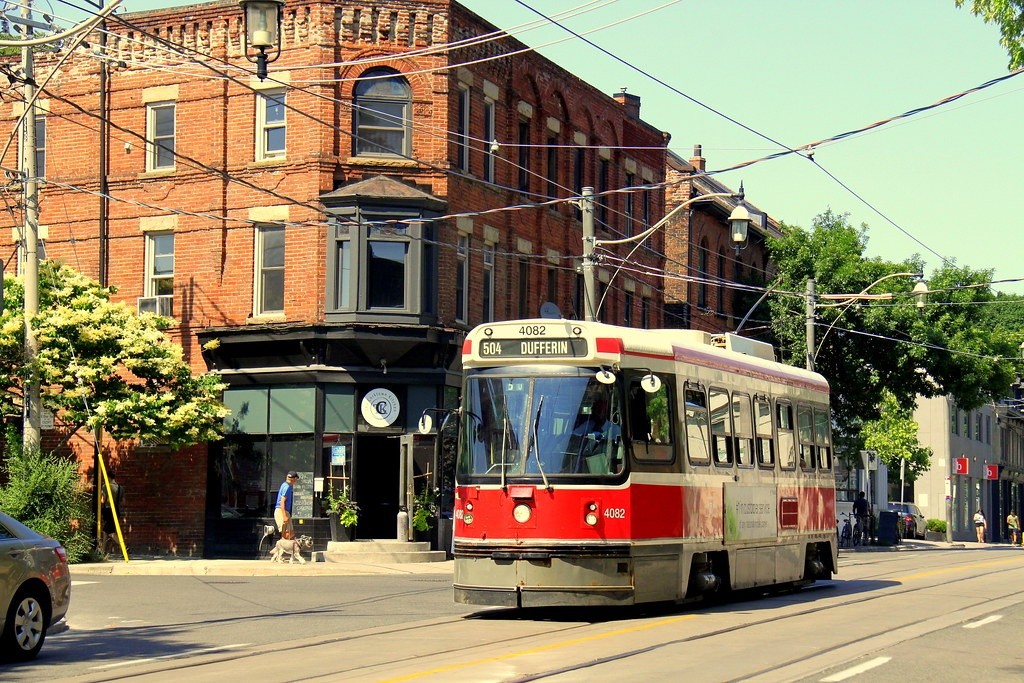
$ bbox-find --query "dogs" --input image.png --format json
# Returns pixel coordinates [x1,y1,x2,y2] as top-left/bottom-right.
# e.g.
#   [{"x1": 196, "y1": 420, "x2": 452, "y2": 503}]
[{"x1": 269, "y1": 534, "x2": 313, "y2": 565}]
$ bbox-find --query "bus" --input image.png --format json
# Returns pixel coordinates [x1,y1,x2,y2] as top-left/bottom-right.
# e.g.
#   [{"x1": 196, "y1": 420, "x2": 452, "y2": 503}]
[{"x1": 417, "y1": 316, "x2": 839, "y2": 613}]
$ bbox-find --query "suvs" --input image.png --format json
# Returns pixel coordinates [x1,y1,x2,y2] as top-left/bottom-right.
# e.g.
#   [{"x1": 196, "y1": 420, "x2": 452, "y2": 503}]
[{"x1": 887, "y1": 500, "x2": 928, "y2": 541}]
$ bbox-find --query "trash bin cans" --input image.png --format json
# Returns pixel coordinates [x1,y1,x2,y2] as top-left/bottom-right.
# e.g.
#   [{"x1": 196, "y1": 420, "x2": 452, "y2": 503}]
[{"x1": 878, "y1": 511, "x2": 898, "y2": 547}]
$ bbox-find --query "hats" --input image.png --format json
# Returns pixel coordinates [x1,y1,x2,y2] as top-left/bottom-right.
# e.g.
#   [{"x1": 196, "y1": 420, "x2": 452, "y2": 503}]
[{"x1": 287, "y1": 470, "x2": 299, "y2": 478}]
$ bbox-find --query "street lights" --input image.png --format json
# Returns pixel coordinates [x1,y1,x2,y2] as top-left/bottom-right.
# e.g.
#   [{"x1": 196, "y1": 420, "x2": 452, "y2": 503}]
[
  {"x1": 803, "y1": 269, "x2": 928, "y2": 375},
  {"x1": 580, "y1": 184, "x2": 753, "y2": 324}
]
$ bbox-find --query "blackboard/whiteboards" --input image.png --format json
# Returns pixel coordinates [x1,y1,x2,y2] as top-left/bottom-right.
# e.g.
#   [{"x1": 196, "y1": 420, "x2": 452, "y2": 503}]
[{"x1": 292, "y1": 472, "x2": 315, "y2": 518}]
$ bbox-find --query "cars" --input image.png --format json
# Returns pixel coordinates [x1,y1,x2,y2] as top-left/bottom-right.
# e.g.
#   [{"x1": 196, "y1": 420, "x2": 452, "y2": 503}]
[{"x1": 0, "y1": 510, "x2": 72, "y2": 663}]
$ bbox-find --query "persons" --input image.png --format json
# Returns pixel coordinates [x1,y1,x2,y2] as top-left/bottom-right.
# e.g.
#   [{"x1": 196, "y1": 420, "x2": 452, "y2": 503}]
[
  {"x1": 274, "y1": 471, "x2": 299, "y2": 540},
  {"x1": 853, "y1": 492, "x2": 870, "y2": 529},
  {"x1": 973, "y1": 510, "x2": 987, "y2": 542},
  {"x1": 570, "y1": 397, "x2": 619, "y2": 441},
  {"x1": 1007, "y1": 510, "x2": 1020, "y2": 544}
]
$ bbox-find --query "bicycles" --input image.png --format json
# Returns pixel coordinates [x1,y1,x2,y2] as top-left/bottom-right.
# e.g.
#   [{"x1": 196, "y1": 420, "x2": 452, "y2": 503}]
[{"x1": 839, "y1": 512, "x2": 865, "y2": 548}]
